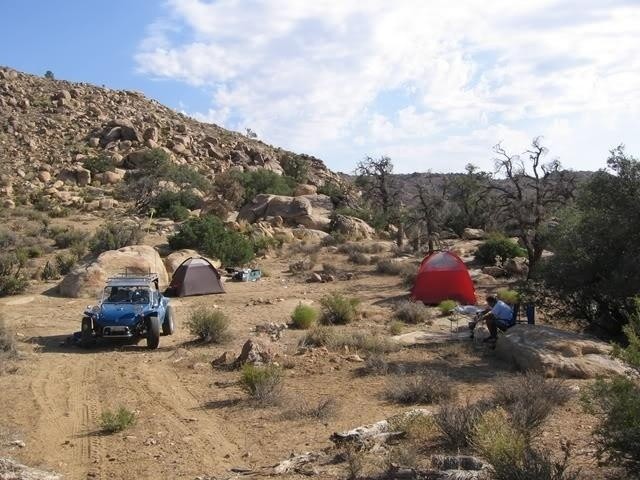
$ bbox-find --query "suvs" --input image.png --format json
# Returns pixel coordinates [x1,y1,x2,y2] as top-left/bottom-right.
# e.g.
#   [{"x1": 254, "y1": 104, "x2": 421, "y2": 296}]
[{"x1": 77, "y1": 266, "x2": 176, "y2": 354}]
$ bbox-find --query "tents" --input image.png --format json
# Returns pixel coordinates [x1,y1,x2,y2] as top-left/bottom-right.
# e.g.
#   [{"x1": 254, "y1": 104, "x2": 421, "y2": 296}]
[
  {"x1": 412, "y1": 250, "x2": 477, "y2": 306},
  {"x1": 170, "y1": 256, "x2": 225, "y2": 296}
]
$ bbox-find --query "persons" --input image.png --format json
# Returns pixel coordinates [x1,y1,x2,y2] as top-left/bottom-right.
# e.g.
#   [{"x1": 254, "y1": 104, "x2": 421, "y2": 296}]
[
  {"x1": 472, "y1": 294, "x2": 514, "y2": 340},
  {"x1": 164, "y1": 278, "x2": 181, "y2": 297}
]
